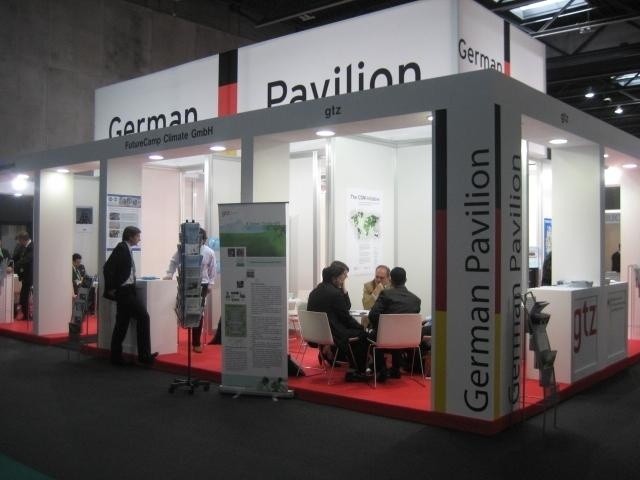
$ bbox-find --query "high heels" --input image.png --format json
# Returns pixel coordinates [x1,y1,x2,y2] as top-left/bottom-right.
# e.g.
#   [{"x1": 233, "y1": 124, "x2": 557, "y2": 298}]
[{"x1": 318, "y1": 352, "x2": 341, "y2": 367}]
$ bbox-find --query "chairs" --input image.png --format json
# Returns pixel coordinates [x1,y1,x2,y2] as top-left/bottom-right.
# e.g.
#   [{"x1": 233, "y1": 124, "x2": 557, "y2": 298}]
[{"x1": 295, "y1": 309, "x2": 426, "y2": 389}]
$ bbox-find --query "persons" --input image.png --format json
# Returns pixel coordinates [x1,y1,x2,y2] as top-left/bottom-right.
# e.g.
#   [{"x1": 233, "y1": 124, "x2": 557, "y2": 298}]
[
  {"x1": 301, "y1": 267, "x2": 363, "y2": 367},
  {"x1": 361, "y1": 262, "x2": 394, "y2": 311},
  {"x1": 72, "y1": 252, "x2": 95, "y2": 315},
  {"x1": 162, "y1": 227, "x2": 216, "y2": 354},
  {"x1": 402, "y1": 317, "x2": 433, "y2": 374},
  {"x1": 102, "y1": 226, "x2": 165, "y2": 371},
  {"x1": 0, "y1": 238, "x2": 12, "y2": 267},
  {"x1": 344, "y1": 265, "x2": 421, "y2": 384},
  {"x1": 316, "y1": 259, "x2": 352, "y2": 367},
  {"x1": 6, "y1": 229, "x2": 33, "y2": 322},
  {"x1": 11, "y1": 233, "x2": 21, "y2": 265}
]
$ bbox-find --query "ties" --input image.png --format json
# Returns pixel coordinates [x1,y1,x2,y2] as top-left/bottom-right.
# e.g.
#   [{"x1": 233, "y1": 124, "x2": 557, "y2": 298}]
[{"x1": 131, "y1": 252, "x2": 136, "y2": 287}]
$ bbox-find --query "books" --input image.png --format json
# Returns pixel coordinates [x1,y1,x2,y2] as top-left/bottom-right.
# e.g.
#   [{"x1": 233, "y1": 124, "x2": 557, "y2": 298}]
[
  {"x1": 173, "y1": 222, "x2": 204, "y2": 328},
  {"x1": 68, "y1": 274, "x2": 97, "y2": 343},
  {"x1": 526, "y1": 300, "x2": 557, "y2": 388}
]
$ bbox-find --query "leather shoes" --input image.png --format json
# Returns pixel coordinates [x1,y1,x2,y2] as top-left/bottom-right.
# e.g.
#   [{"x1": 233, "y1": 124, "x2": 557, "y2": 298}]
[
  {"x1": 141, "y1": 352, "x2": 158, "y2": 367},
  {"x1": 349, "y1": 368, "x2": 400, "y2": 382},
  {"x1": 194, "y1": 345, "x2": 202, "y2": 353}
]
